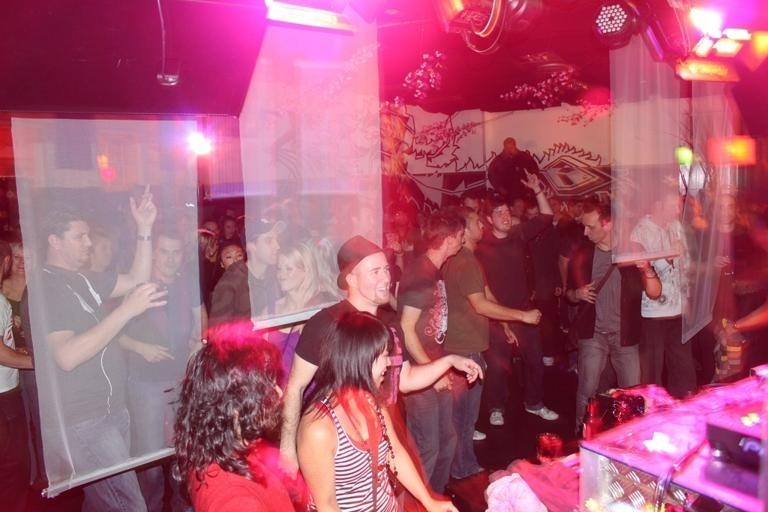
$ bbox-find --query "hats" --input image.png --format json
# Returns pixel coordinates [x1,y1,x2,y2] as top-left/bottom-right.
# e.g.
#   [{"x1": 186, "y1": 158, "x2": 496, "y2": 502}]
[
  {"x1": 242, "y1": 218, "x2": 285, "y2": 247},
  {"x1": 337, "y1": 235, "x2": 394, "y2": 291}
]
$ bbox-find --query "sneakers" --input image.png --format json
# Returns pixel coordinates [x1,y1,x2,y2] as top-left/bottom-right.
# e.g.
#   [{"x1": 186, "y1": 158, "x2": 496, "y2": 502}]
[
  {"x1": 490, "y1": 411, "x2": 504, "y2": 425},
  {"x1": 526, "y1": 407, "x2": 559, "y2": 421},
  {"x1": 471, "y1": 430, "x2": 487, "y2": 440}
]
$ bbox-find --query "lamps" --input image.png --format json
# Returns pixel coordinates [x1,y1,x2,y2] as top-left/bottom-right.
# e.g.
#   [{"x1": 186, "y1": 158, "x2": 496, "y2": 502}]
[{"x1": 591, "y1": 2, "x2": 650, "y2": 50}]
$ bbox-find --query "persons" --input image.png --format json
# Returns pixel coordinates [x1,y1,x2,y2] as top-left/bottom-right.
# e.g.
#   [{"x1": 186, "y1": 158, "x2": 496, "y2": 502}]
[
  {"x1": 174, "y1": 337, "x2": 309, "y2": 510},
  {"x1": 393, "y1": 211, "x2": 468, "y2": 491},
  {"x1": 630, "y1": 189, "x2": 767, "y2": 400},
  {"x1": 196, "y1": 197, "x2": 422, "y2": 389},
  {"x1": 442, "y1": 166, "x2": 581, "y2": 479},
  {"x1": 280, "y1": 234, "x2": 483, "y2": 506},
  {"x1": 1, "y1": 184, "x2": 217, "y2": 510},
  {"x1": 566, "y1": 197, "x2": 662, "y2": 433},
  {"x1": 297, "y1": 311, "x2": 458, "y2": 512}
]
